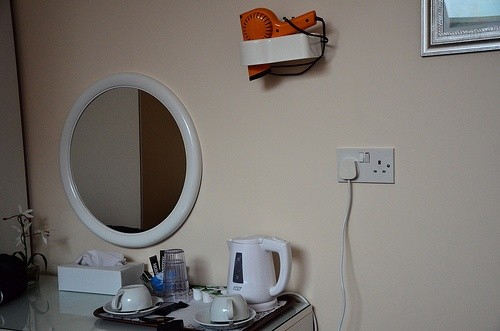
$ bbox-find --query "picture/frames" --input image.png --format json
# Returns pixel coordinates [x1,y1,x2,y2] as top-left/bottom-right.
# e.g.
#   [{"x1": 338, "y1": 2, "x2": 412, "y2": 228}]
[{"x1": 421, "y1": 0, "x2": 500, "y2": 57}]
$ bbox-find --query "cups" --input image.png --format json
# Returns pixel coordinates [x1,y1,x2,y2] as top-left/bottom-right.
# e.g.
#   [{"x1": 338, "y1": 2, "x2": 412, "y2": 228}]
[
  {"x1": 193, "y1": 287, "x2": 222, "y2": 304},
  {"x1": 163, "y1": 248, "x2": 190, "y2": 303},
  {"x1": 112, "y1": 284, "x2": 153, "y2": 312},
  {"x1": 211, "y1": 294, "x2": 249, "y2": 322}
]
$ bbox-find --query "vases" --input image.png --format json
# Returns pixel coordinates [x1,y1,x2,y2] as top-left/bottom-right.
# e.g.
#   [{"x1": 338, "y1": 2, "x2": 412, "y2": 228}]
[{"x1": 16, "y1": 263, "x2": 40, "y2": 288}]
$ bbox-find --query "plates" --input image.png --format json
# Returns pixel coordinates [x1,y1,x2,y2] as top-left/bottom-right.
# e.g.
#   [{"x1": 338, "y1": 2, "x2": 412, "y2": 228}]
[
  {"x1": 193, "y1": 308, "x2": 256, "y2": 327},
  {"x1": 103, "y1": 296, "x2": 164, "y2": 315}
]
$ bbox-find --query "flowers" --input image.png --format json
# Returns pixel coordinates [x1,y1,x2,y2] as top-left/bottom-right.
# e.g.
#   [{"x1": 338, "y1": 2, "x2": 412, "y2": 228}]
[{"x1": 3, "y1": 200, "x2": 52, "y2": 273}]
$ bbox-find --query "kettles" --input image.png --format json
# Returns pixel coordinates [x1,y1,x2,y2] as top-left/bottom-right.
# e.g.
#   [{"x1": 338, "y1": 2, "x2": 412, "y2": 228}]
[{"x1": 226, "y1": 236, "x2": 292, "y2": 312}]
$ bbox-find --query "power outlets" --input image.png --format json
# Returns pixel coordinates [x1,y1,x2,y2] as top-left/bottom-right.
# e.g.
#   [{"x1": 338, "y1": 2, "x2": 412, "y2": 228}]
[{"x1": 335, "y1": 147, "x2": 395, "y2": 183}]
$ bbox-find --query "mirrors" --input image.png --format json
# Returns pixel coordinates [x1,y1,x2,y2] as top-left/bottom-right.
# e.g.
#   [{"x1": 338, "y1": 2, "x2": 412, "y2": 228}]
[{"x1": 59, "y1": 71, "x2": 202, "y2": 248}]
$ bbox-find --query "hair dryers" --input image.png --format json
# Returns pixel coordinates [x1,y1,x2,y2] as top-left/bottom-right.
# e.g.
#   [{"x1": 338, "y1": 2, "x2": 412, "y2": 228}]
[{"x1": 238, "y1": 4, "x2": 317, "y2": 82}]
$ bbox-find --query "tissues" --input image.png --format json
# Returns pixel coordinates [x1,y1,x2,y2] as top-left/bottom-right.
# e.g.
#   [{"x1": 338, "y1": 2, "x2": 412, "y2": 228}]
[{"x1": 57, "y1": 250, "x2": 147, "y2": 297}]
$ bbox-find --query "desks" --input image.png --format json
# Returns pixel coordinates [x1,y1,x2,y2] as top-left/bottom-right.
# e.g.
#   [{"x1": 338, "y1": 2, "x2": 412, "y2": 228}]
[{"x1": 0, "y1": 262, "x2": 315, "y2": 331}]
